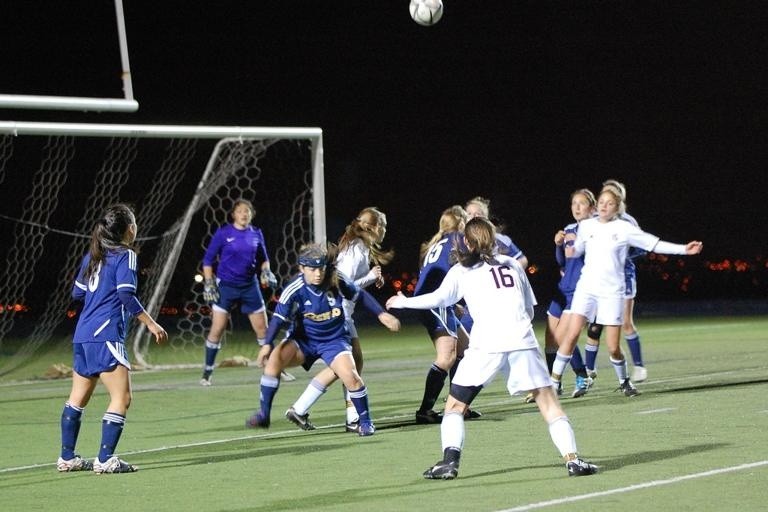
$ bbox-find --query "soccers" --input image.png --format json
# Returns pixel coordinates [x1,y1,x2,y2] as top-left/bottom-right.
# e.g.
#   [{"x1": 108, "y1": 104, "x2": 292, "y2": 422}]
[{"x1": 410, "y1": 0, "x2": 444, "y2": 26}]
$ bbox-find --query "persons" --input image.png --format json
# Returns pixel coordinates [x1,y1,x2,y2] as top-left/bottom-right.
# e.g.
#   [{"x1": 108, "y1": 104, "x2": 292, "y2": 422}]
[
  {"x1": 525, "y1": 179, "x2": 703, "y2": 403},
  {"x1": 198, "y1": 197, "x2": 296, "y2": 386},
  {"x1": 701, "y1": 250, "x2": 768, "y2": 301},
  {"x1": 54, "y1": 201, "x2": 169, "y2": 475},
  {"x1": 385, "y1": 214, "x2": 598, "y2": 480},
  {"x1": 259, "y1": 273, "x2": 286, "y2": 312},
  {"x1": 284, "y1": 206, "x2": 387, "y2": 433},
  {"x1": 414, "y1": 197, "x2": 529, "y2": 424},
  {"x1": 380, "y1": 264, "x2": 424, "y2": 300},
  {"x1": 0, "y1": 300, "x2": 34, "y2": 339},
  {"x1": 244, "y1": 241, "x2": 401, "y2": 437},
  {"x1": 61, "y1": 307, "x2": 79, "y2": 333},
  {"x1": 157, "y1": 300, "x2": 214, "y2": 319}
]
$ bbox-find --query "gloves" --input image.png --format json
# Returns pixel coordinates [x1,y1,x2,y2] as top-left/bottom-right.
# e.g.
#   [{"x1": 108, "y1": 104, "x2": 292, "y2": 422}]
[
  {"x1": 203, "y1": 278, "x2": 220, "y2": 303},
  {"x1": 260, "y1": 268, "x2": 278, "y2": 289}
]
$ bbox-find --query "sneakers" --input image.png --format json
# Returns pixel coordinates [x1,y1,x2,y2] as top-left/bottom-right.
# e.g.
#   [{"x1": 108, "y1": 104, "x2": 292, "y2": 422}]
[
  {"x1": 585, "y1": 367, "x2": 596, "y2": 381},
  {"x1": 201, "y1": 370, "x2": 212, "y2": 386},
  {"x1": 345, "y1": 419, "x2": 359, "y2": 431},
  {"x1": 422, "y1": 462, "x2": 458, "y2": 481},
  {"x1": 567, "y1": 460, "x2": 598, "y2": 476},
  {"x1": 57, "y1": 455, "x2": 93, "y2": 472},
  {"x1": 93, "y1": 456, "x2": 138, "y2": 474},
  {"x1": 464, "y1": 410, "x2": 481, "y2": 419},
  {"x1": 572, "y1": 376, "x2": 594, "y2": 397},
  {"x1": 524, "y1": 381, "x2": 562, "y2": 404},
  {"x1": 358, "y1": 419, "x2": 375, "y2": 437},
  {"x1": 246, "y1": 413, "x2": 271, "y2": 429},
  {"x1": 280, "y1": 372, "x2": 296, "y2": 381},
  {"x1": 619, "y1": 378, "x2": 637, "y2": 398},
  {"x1": 414, "y1": 408, "x2": 443, "y2": 423},
  {"x1": 284, "y1": 407, "x2": 317, "y2": 430},
  {"x1": 632, "y1": 366, "x2": 647, "y2": 382}
]
